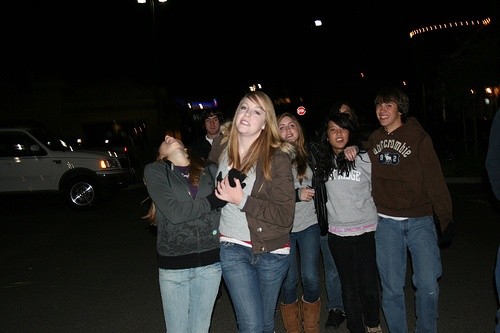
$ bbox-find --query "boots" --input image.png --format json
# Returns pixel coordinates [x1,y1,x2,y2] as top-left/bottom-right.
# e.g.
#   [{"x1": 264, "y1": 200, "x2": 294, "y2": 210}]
[
  {"x1": 301, "y1": 295, "x2": 322, "y2": 333},
  {"x1": 279, "y1": 299, "x2": 301, "y2": 333}
]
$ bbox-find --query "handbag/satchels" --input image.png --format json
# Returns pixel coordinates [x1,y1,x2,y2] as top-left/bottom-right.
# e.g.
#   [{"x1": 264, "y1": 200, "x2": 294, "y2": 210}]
[{"x1": 310, "y1": 151, "x2": 329, "y2": 237}]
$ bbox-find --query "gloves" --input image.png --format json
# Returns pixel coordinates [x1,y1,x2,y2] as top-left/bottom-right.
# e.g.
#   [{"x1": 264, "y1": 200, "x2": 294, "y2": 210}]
[{"x1": 207, "y1": 169, "x2": 247, "y2": 211}]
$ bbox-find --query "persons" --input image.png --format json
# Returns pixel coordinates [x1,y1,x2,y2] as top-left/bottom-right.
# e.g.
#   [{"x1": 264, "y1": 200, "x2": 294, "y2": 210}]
[
  {"x1": 143, "y1": 136, "x2": 246, "y2": 333},
  {"x1": 196, "y1": 88, "x2": 500, "y2": 333}
]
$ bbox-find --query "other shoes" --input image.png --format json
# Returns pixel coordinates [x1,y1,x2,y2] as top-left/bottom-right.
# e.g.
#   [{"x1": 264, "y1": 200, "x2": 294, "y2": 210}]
[
  {"x1": 325, "y1": 309, "x2": 347, "y2": 331},
  {"x1": 367, "y1": 324, "x2": 382, "y2": 333}
]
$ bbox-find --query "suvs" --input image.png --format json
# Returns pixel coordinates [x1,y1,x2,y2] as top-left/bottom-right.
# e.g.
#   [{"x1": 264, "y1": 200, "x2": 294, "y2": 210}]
[{"x1": 0, "y1": 125, "x2": 135, "y2": 212}]
